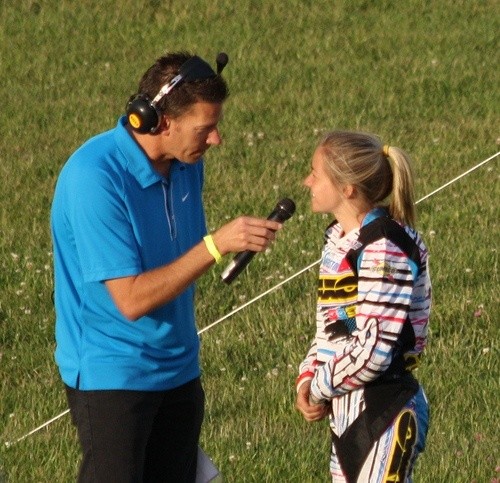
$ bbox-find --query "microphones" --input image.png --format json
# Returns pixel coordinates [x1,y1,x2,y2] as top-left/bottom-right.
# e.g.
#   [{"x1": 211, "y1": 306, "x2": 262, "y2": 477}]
[
  {"x1": 220, "y1": 199, "x2": 298, "y2": 284},
  {"x1": 215, "y1": 55, "x2": 229, "y2": 75}
]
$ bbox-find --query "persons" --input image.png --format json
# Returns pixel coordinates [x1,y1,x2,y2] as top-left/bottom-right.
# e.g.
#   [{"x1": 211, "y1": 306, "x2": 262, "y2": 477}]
[
  {"x1": 294, "y1": 129, "x2": 433, "y2": 483},
  {"x1": 50, "y1": 50, "x2": 283, "y2": 483}
]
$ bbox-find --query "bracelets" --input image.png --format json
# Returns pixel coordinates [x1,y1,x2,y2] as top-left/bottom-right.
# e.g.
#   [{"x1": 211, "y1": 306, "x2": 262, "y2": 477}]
[{"x1": 202, "y1": 235, "x2": 224, "y2": 265}]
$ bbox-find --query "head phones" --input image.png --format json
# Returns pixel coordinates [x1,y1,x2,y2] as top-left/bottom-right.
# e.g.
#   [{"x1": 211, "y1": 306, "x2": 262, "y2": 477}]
[{"x1": 125, "y1": 61, "x2": 214, "y2": 135}]
